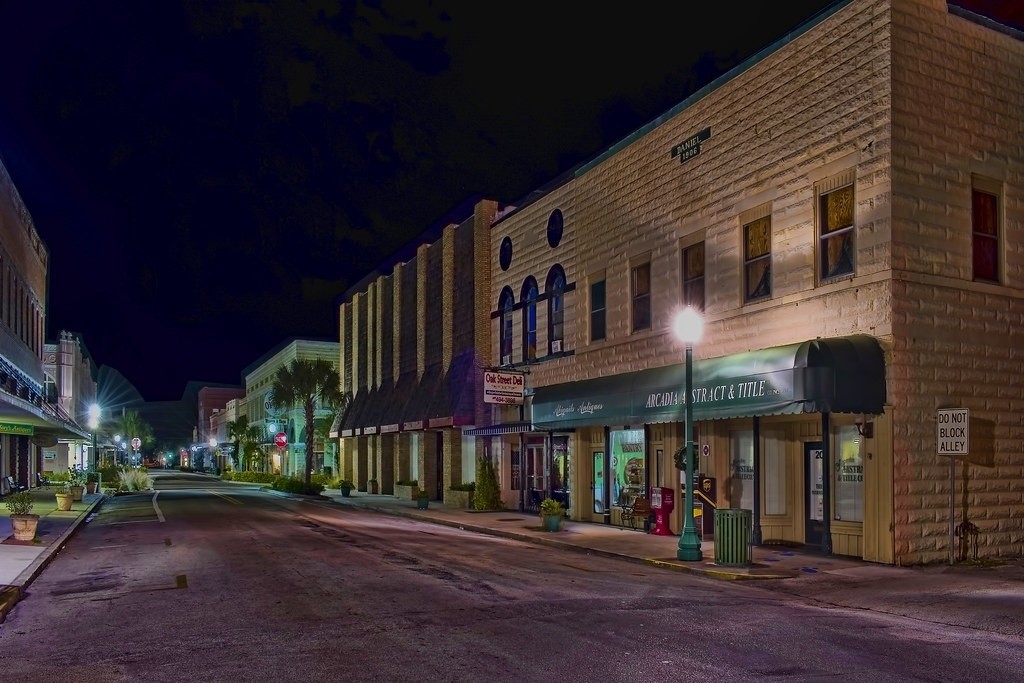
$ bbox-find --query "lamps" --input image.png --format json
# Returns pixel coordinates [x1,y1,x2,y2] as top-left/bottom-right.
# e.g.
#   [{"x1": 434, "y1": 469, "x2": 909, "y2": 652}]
[{"x1": 854, "y1": 418, "x2": 875, "y2": 440}]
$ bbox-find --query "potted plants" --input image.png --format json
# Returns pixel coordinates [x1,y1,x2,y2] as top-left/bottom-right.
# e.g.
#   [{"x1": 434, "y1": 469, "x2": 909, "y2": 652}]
[
  {"x1": 66, "y1": 463, "x2": 86, "y2": 501},
  {"x1": 338, "y1": 478, "x2": 356, "y2": 497},
  {"x1": 1, "y1": 488, "x2": 40, "y2": 542},
  {"x1": 417, "y1": 491, "x2": 429, "y2": 510},
  {"x1": 53, "y1": 481, "x2": 74, "y2": 511},
  {"x1": 539, "y1": 499, "x2": 566, "y2": 532},
  {"x1": 85, "y1": 472, "x2": 100, "y2": 494}
]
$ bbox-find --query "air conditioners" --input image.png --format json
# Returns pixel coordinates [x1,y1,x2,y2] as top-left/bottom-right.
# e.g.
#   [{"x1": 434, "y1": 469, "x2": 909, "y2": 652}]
[
  {"x1": 502, "y1": 354, "x2": 512, "y2": 365},
  {"x1": 551, "y1": 339, "x2": 562, "y2": 353}
]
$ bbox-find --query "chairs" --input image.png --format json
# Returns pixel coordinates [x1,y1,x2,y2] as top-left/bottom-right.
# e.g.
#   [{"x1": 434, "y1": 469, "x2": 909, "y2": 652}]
[{"x1": 525, "y1": 486, "x2": 542, "y2": 513}]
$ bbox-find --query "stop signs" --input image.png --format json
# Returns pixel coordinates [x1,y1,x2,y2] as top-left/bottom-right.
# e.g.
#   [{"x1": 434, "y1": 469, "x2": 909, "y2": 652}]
[{"x1": 274, "y1": 432, "x2": 288, "y2": 447}]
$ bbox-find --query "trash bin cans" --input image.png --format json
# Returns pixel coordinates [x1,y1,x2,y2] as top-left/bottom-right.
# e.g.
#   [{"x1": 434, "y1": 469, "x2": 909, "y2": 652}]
[
  {"x1": 713, "y1": 507, "x2": 753, "y2": 568},
  {"x1": 87, "y1": 472, "x2": 102, "y2": 492},
  {"x1": 324, "y1": 466, "x2": 331, "y2": 479},
  {"x1": 212, "y1": 468, "x2": 221, "y2": 476}
]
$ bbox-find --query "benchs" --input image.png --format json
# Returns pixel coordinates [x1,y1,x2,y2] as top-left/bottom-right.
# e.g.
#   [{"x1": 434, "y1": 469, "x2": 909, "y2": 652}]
[
  {"x1": 620, "y1": 496, "x2": 651, "y2": 532},
  {"x1": 36, "y1": 472, "x2": 51, "y2": 491},
  {"x1": 6, "y1": 476, "x2": 25, "y2": 494}
]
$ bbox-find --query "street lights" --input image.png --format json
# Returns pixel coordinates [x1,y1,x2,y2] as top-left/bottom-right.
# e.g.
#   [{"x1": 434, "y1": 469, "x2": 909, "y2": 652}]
[
  {"x1": 89, "y1": 404, "x2": 100, "y2": 472},
  {"x1": 114, "y1": 434, "x2": 120, "y2": 467},
  {"x1": 209, "y1": 438, "x2": 217, "y2": 474},
  {"x1": 672, "y1": 307, "x2": 706, "y2": 561}
]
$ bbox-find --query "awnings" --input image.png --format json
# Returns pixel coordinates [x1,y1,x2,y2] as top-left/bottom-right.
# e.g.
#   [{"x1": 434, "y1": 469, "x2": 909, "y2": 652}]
[
  {"x1": 328, "y1": 352, "x2": 475, "y2": 438},
  {"x1": 530, "y1": 333, "x2": 886, "y2": 431},
  {"x1": 462, "y1": 423, "x2": 575, "y2": 436}
]
